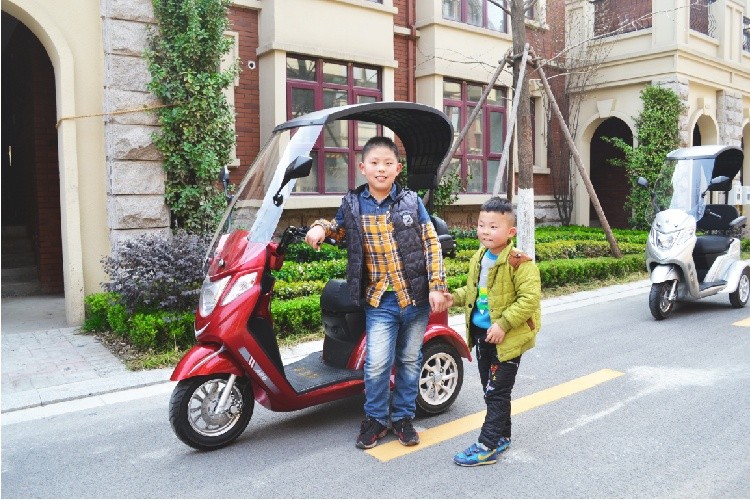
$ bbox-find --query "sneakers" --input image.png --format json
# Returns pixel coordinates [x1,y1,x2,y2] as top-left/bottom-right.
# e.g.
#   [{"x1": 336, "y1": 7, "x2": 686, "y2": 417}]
[
  {"x1": 496, "y1": 436, "x2": 511, "y2": 452},
  {"x1": 356, "y1": 416, "x2": 388, "y2": 449},
  {"x1": 451, "y1": 441, "x2": 496, "y2": 466},
  {"x1": 392, "y1": 415, "x2": 420, "y2": 445}
]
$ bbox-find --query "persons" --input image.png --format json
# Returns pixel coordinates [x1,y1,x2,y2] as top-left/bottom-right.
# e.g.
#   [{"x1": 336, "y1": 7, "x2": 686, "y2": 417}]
[
  {"x1": 304, "y1": 136, "x2": 449, "y2": 448},
  {"x1": 443, "y1": 196, "x2": 543, "y2": 468}
]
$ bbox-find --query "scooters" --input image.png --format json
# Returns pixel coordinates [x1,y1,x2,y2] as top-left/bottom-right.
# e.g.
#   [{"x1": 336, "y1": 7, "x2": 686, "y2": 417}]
[
  {"x1": 637, "y1": 143, "x2": 750, "y2": 320},
  {"x1": 162, "y1": 100, "x2": 476, "y2": 456}
]
